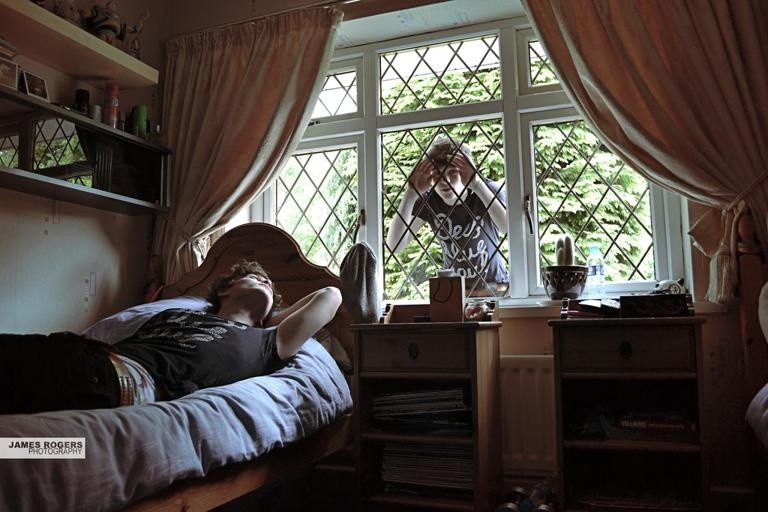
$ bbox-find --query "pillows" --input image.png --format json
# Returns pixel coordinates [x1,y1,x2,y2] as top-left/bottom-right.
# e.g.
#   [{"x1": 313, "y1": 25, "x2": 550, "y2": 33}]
[{"x1": 271, "y1": 299, "x2": 352, "y2": 374}]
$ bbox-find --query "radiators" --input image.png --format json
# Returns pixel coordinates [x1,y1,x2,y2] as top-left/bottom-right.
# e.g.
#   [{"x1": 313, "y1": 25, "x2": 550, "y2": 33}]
[{"x1": 495, "y1": 354, "x2": 556, "y2": 479}]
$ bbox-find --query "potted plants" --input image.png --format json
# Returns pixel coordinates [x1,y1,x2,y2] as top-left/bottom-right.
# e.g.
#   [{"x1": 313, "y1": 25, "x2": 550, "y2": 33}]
[{"x1": 542, "y1": 236, "x2": 588, "y2": 300}]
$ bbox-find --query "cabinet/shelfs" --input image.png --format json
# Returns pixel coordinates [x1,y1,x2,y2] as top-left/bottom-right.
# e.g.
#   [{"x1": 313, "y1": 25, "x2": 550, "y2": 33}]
[
  {"x1": 544, "y1": 314, "x2": 708, "y2": 512},
  {"x1": 0, "y1": 83, "x2": 173, "y2": 216},
  {"x1": 347, "y1": 320, "x2": 503, "y2": 512}
]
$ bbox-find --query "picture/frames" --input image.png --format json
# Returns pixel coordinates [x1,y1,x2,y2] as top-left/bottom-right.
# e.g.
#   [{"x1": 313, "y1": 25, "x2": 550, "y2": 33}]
[
  {"x1": 0, "y1": 57, "x2": 18, "y2": 91},
  {"x1": 22, "y1": 69, "x2": 50, "y2": 103}
]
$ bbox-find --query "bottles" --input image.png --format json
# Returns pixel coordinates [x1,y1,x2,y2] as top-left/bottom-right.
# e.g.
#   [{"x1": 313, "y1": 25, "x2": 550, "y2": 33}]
[
  {"x1": 104, "y1": 82, "x2": 120, "y2": 130},
  {"x1": 91, "y1": 105, "x2": 101, "y2": 122},
  {"x1": 587, "y1": 245, "x2": 606, "y2": 298},
  {"x1": 119, "y1": 111, "x2": 127, "y2": 133},
  {"x1": 131, "y1": 104, "x2": 148, "y2": 139}
]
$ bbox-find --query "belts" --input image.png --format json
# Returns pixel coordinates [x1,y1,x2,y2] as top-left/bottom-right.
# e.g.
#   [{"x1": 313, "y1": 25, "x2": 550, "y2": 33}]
[{"x1": 107, "y1": 351, "x2": 134, "y2": 405}]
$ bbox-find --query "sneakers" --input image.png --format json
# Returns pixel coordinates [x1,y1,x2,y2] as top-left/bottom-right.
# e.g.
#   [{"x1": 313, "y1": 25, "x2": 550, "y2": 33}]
[
  {"x1": 497, "y1": 485, "x2": 527, "y2": 511},
  {"x1": 529, "y1": 485, "x2": 554, "y2": 511}
]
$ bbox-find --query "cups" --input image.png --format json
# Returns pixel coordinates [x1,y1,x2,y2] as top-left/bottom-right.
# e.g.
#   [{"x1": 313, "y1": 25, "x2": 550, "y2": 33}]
[{"x1": 437, "y1": 270, "x2": 453, "y2": 277}]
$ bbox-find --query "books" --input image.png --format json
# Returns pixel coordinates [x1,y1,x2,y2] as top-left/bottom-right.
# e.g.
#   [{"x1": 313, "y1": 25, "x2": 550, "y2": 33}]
[{"x1": 373, "y1": 389, "x2": 475, "y2": 500}]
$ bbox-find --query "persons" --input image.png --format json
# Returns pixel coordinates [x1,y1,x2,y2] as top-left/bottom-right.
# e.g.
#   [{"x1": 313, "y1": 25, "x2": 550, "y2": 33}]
[
  {"x1": 386, "y1": 137, "x2": 508, "y2": 296},
  {"x1": 0, "y1": 256, "x2": 342, "y2": 415}
]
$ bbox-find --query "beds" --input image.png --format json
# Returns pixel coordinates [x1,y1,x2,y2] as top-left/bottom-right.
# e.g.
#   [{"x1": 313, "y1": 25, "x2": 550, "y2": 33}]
[
  {"x1": 736, "y1": 211, "x2": 768, "y2": 512},
  {"x1": 0, "y1": 219, "x2": 357, "y2": 512}
]
